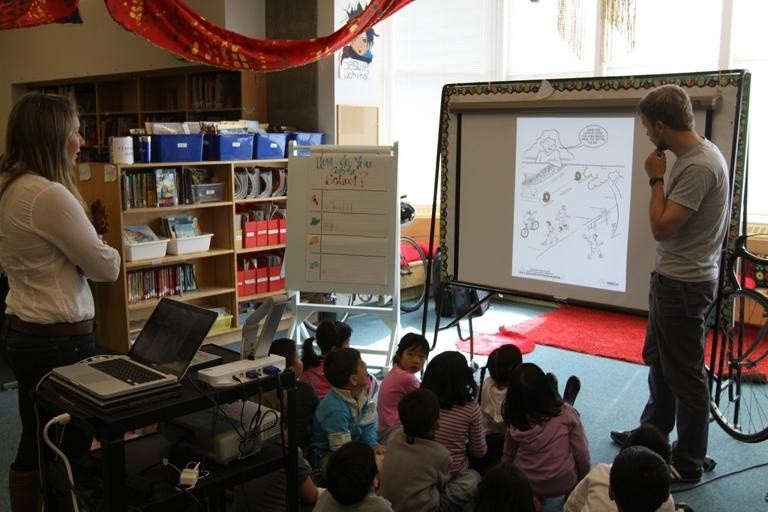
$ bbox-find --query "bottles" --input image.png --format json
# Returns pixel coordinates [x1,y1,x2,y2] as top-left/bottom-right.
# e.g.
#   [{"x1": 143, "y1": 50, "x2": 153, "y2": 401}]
[{"x1": 138, "y1": 135, "x2": 153, "y2": 162}]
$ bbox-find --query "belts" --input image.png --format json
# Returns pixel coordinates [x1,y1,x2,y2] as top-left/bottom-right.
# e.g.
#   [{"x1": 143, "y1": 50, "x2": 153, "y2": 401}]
[{"x1": 3, "y1": 314, "x2": 95, "y2": 336}]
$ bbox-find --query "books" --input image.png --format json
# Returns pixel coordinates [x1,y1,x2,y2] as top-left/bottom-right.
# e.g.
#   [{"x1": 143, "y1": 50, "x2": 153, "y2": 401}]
[
  {"x1": 111, "y1": 119, "x2": 286, "y2": 165},
  {"x1": 233, "y1": 168, "x2": 288, "y2": 200},
  {"x1": 237, "y1": 254, "x2": 288, "y2": 298},
  {"x1": 123, "y1": 167, "x2": 226, "y2": 210},
  {"x1": 127, "y1": 264, "x2": 199, "y2": 302},
  {"x1": 233, "y1": 203, "x2": 287, "y2": 249},
  {"x1": 122, "y1": 215, "x2": 214, "y2": 262}
]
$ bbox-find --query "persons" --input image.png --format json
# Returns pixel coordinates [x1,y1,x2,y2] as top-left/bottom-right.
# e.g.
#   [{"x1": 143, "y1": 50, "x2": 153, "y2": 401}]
[
  {"x1": 269, "y1": 316, "x2": 677, "y2": 512},
  {"x1": 610, "y1": 84, "x2": 730, "y2": 484},
  {"x1": 0, "y1": 93, "x2": 121, "y2": 511}
]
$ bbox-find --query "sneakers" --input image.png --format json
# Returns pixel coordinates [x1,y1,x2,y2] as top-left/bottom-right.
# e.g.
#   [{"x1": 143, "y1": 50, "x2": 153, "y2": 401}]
[
  {"x1": 611, "y1": 427, "x2": 642, "y2": 444},
  {"x1": 547, "y1": 372, "x2": 580, "y2": 404},
  {"x1": 666, "y1": 461, "x2": 703, "y2": 484}
]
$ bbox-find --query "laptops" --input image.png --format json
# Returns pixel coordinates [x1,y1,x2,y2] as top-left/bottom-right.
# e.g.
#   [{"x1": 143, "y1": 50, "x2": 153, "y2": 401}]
[{"x1": 52, "y1": 295, "x2": 219, "y2": 403}]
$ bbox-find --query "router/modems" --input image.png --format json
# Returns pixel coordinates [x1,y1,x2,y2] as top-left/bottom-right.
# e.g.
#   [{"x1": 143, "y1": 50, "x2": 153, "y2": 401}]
[{"x1": 198, "y1": 352, "x2": 285, "y2": 386}]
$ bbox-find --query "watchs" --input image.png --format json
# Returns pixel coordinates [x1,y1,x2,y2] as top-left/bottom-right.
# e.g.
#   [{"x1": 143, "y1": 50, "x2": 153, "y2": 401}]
[{"x1": 649, "y1": 176, "x2": 664, "y2": 186}]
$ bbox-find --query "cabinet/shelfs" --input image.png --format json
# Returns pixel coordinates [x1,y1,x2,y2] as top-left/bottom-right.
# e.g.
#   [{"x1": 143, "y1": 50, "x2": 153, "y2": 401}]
[
  {"x1": 76, "y1": 163, "x2": 326, "y2": 354},
  {"x1": 27, "y1": 344, "x2": 302, "y2": 512},
  {"x1": 9, "y1": 65, "x2": 242, "y2": 163}
]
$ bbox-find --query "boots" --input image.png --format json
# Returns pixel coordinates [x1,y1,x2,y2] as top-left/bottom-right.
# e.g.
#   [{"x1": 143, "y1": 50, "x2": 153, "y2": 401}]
[{"x1": 8, "y1": 463, "x2": 44, "y2": 512}]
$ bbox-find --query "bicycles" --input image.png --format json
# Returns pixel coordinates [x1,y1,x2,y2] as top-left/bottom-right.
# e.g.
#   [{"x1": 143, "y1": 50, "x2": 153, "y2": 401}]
[
  {"x1": 703, "y1": 234, "x2": 768, "y2": 444},
  {"x1": 291, "y1": 196, "x2": 429, "y2": 332}
]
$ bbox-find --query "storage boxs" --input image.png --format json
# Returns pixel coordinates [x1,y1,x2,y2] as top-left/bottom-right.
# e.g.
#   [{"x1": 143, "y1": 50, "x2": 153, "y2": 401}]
[
  {"x1": 248, "y1": 132, "x2": 292, "y2": 160},
  {"x1": 131, "y1": 133, "x2": 204, "y2": 164},
  {"x1": 284, "y1": 129, "x2": 324, "y2": 156},
  {"x1": 201, "y1": 134, "x2": 254, "y2": 162}
]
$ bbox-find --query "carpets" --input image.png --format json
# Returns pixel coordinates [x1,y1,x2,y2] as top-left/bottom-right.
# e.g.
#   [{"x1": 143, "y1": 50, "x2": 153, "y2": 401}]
[
  {"x1": 456, "y1": 326, "x2": 535, "y2": 355},
  {"x1": 508, "y1": 304, "x2": 768, "y2": 383}
]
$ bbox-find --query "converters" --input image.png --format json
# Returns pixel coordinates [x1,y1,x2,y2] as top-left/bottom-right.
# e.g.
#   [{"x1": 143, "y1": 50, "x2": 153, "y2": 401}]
[{"x1": 180, "y1": 468, "x2": 198, "y2": 486}]
